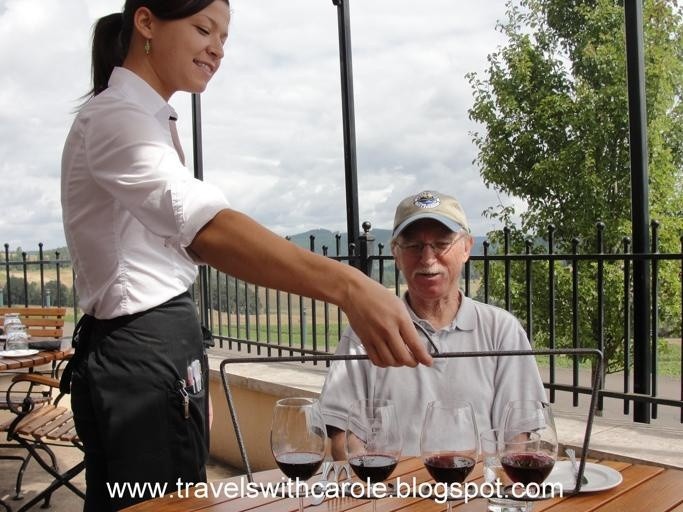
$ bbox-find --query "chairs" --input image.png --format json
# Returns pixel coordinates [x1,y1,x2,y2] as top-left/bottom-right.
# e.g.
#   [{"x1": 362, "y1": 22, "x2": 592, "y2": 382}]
[
  {"x1": 6, "y1": 350, "x2": 87, "y2": 512},
  {"x1": 0, "y1": 308, "x2": 65, "y2": 411}
]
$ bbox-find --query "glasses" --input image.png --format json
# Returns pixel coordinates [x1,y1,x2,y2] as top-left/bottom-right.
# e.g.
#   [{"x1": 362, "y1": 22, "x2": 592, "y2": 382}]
[{"x1": 394, "y1": 232, "x2": 465, "y2": 253}]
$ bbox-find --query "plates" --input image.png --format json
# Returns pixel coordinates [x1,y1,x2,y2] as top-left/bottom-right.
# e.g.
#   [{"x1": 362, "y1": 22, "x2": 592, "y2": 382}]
[
  {"x1": 0, "y1": 349, "x2": 40, "y2": 358},
  {"x1": 541, "y1": 459, "x2": 623, "y2": 494}
]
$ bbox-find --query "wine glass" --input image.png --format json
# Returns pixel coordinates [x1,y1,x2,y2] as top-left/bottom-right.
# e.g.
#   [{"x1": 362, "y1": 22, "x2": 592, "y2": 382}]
[
  {"x1": 270, "y1": 398, "x2": 328, "y2": 512},
  {"x1": 497, "y1": 400, "x2": 558, "y2": 511},
  {"x1": 343, "y1": 398, "x2": 402, "y2": 512}
]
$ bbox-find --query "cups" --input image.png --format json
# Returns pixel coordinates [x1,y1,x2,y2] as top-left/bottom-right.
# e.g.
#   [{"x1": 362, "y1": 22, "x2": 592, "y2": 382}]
[
  {"x1": 481, "y1": 430, "x2": 540, "y2": 511},
  {"x1": 5, "y1": 325, "x2": 29, "y2": 352},
  {"x1": 420, "y1": 400, "x2": 479, "y2": 512},
  {"x1": 3, "y1": 313, "x2": 20, "y2": 336}
]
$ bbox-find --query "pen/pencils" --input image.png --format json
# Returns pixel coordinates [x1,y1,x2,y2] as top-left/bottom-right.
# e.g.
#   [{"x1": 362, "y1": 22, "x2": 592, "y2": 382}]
[{"x1": 187, "y1": 359, "x2": 203, "y2": 393}]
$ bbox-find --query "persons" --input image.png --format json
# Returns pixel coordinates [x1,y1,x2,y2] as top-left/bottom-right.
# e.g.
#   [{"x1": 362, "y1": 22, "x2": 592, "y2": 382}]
[
  {"x1": 60, "y1": 1, "x2": 432, "y2": 512},
  {"x1": 308, "y1": 188, "x2": 550, "y2": 462}
]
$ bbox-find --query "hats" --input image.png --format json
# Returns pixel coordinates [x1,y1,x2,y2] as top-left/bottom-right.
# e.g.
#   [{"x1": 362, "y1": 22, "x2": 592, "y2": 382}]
[{"x1": 391, "y1": 191, "x2": 470, "y2": 240}]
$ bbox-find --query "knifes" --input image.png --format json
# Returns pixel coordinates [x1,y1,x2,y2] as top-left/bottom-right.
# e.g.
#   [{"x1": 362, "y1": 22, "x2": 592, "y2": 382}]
[{"x1": 565, "y1": 448, "x2": 589, "y2": 484}]
